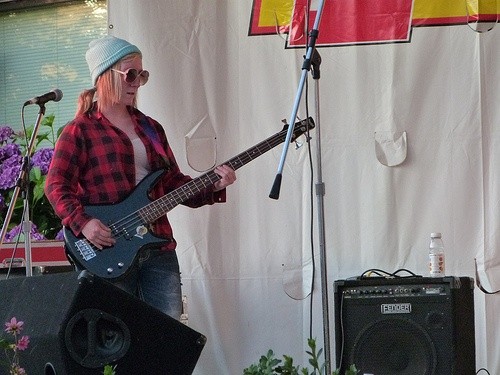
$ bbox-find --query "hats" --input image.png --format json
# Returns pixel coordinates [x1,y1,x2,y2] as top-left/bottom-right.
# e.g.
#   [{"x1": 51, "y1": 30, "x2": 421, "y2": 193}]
[{"x1": 85, "y1": 36, "x2": 141, "y2": 88}]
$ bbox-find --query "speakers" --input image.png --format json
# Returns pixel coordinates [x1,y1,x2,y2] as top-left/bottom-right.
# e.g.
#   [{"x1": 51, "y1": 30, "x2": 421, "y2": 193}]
[
  {"x1": 0, "y1": 272, "x2": 208, "y2": 375},
  {"x1": 332, "y1": 276, "x2": 477, "y2": 375}
]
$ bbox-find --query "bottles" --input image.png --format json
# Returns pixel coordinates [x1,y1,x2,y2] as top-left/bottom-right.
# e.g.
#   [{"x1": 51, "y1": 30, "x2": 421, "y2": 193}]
[{"x1": 429, "y1": 232, "x2": 446, "y2": 278}]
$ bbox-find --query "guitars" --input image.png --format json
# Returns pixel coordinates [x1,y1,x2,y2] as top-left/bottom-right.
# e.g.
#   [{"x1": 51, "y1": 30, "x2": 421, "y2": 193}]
[{"x1": 64, "y1": 114, "x2": 317, "y2": 281}]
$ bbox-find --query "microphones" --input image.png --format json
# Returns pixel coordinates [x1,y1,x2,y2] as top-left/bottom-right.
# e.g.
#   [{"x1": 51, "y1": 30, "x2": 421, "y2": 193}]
[{"x1": 24, "y1": 88, "x2": 63, "y2": 106}]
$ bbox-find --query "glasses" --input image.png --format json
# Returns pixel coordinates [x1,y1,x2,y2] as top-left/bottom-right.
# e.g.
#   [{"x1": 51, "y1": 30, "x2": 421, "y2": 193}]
[{"x1": 111, "y1": 68, "x2": 149, "y2": 86}]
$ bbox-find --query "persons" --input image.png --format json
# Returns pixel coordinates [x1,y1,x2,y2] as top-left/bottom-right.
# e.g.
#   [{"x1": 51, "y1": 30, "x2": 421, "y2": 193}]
[{"x1": 43, "y1": 37, "x2": 238, "y2": 323}]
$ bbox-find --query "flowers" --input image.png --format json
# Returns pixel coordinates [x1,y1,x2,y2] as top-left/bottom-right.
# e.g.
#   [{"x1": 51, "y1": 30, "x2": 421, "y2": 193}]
[
  {"x1": 0, "y1": 315, "x2": 31, "y2": 375},
  {"x1": 0, "y1": 111, "x2": 65, "y2": 242}
]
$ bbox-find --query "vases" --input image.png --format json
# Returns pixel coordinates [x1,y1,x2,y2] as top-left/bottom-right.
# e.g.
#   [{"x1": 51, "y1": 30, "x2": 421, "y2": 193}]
[{"x1": 0, "y1": 243, "x2": 71, "y2": 269}]
